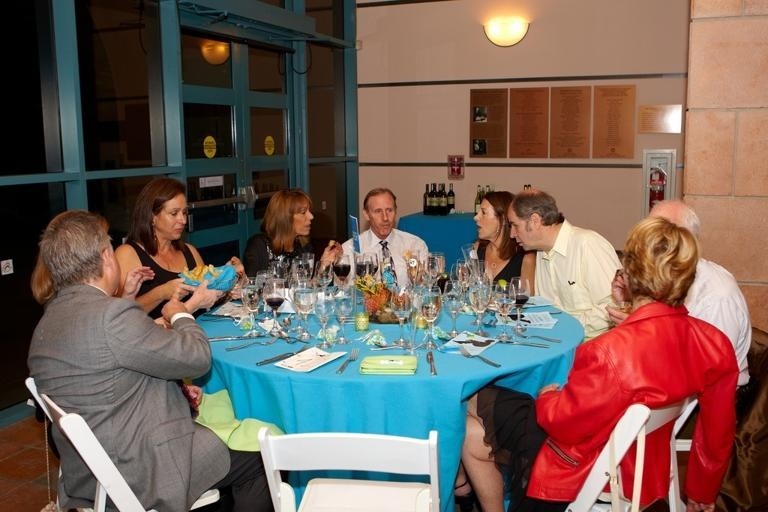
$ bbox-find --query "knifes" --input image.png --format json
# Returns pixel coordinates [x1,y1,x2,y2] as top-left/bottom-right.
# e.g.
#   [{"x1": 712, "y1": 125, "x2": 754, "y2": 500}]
[{"x1": 424, "y1": 350, "x2": 438, "y2": 378}]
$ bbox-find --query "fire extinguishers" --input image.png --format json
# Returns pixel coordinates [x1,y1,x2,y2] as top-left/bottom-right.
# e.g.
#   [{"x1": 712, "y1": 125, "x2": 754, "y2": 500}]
[{"x1": 649, "y1": 163, "x2": 668, "y2": 208}]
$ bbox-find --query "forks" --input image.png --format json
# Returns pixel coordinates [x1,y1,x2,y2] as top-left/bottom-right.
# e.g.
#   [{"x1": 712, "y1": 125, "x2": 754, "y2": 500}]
[
  {"x1": 512, "y1": 326, "x2": 567, "y2": 344},
  {"x1": 336, "y1": 348, "x2": 358, "y2": 374},
  {"x1": 223, "y1": 334, "x2": 281, "y2": 352},
  {"x1": 254, "y1": 347, "x2": 308, "y2": 367},
  {"x1": 460, "y1": 347, "x2": 501, "y2": 368}
]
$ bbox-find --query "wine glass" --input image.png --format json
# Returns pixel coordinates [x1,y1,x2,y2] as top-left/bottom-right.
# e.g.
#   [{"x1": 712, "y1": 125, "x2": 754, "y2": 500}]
[{"x1": 243, "y1": 252, "x2": 531, "y2": 349}]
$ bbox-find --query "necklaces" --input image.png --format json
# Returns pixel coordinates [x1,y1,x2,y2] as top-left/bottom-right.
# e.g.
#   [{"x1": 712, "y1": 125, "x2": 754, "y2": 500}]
[{"x1": 488, "y1": 246, "x2": 503, "y2": 269}]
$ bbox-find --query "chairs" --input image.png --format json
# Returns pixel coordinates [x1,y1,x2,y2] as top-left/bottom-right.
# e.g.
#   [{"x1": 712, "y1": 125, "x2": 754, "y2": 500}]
[
  {"x1": 255, "y1": 423, "x2": 443, "y2": 511},
  {"x1": 21, "y1": 378, "x2": 222, "y2": 511},
  {"x1": 676, "y1": 329, "x2": 765, "y2": 512},
  {"x1": 565, "y1": 394, "x2": 696, "y2": 512}
]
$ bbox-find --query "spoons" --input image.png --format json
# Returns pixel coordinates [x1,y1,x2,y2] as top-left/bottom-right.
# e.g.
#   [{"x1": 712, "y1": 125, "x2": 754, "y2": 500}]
[{"x1": 203, "y1": 329, "x2": 263, "y2": 340}]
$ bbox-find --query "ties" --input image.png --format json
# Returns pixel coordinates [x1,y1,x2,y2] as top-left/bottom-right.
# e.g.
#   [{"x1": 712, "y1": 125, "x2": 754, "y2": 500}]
[{"x1": 379, "y1": 240, "x2": 398, "y2": 286}]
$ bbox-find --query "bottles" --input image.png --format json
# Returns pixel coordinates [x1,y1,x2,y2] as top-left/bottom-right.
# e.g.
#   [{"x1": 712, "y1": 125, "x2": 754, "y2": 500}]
[
  {"x1": 474, "y1": 184, "x2": 492, "y2": 215},
  {"x1": 421, "y1": 182, "x2": 455, "y2": 216}
]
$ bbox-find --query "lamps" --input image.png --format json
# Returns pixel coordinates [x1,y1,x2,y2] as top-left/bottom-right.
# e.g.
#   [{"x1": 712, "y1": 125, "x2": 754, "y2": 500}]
[
  {"x1": 481, "y1": 12, "x2": 532, "y2": 49},
  {"x1": 202, "y1": 39, "x2": 229, "y2": 67}
]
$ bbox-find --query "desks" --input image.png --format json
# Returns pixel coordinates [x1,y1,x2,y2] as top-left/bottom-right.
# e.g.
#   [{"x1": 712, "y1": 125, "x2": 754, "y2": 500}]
[{"x1": 396, "y1": 212, "x2": 479, "y2": 280}]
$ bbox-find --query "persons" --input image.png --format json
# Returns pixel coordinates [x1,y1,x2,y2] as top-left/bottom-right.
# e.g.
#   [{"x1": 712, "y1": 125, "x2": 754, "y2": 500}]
[
  {"x1": 507, "y1": 187, "x2": 627, "y2": 343},
  {"x1": 335, "y1": 187, "x2": 430, "y2": 295},
  {"x1": 24, "y1": 208, "x2": 289, "y2": 512},
  {"x1": 114, "y1": 176, "x2": 250, "y2": 316},
  {"x1": 29, "y1": 247, "x2": 226, "y2": 459},
  {"x1": 453, "y1": 216, "x2": 742, "y2": 512},
  {"x1": 465, "y1": 189, "x2": 537, "y2": 299},
  {"x1": 604, "y1": 197, "x2": 754, "y2": 435},
  {"x1": 243, "y1": 186, "x2": 344, "y2": 288}
]
